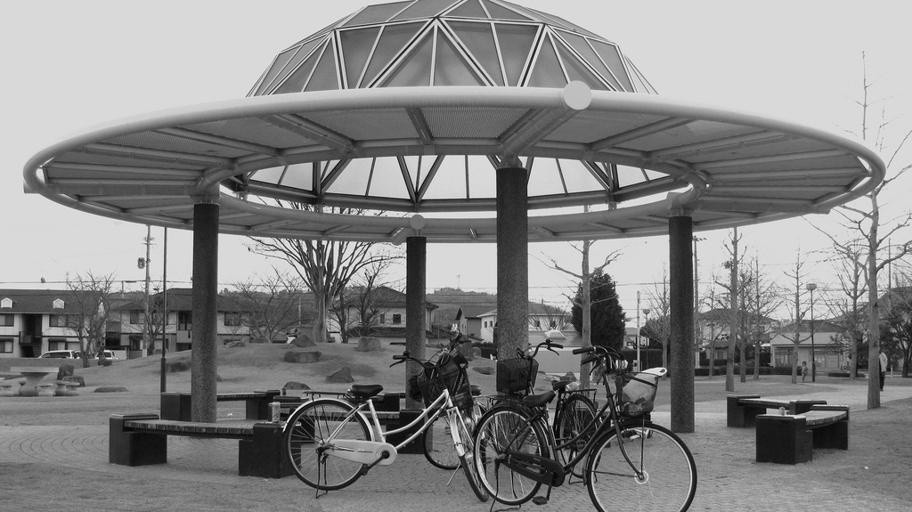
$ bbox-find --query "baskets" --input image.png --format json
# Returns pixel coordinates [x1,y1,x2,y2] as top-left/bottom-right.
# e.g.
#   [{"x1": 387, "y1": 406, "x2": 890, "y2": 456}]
[
  {"x1": 617, "y1": 371, "x2": 657, "y2": 415},
  {"x1": 496, "y1": 356, "x2": 539, "y2": 393},
  {"x1": 418, "y1": 369, "x2": 473, "y2": 420}
]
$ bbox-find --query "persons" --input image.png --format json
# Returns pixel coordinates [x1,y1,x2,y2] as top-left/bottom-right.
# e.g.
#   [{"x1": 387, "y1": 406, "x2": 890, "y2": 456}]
[
  {"x1": 802, "y1": 361, "x2": 808, "y2": 382},
  {"x1": 96, "y1": 342, "x2": 105, "y2": 357},
  {"x1": 879, "y1": 352, "x2": 888, "y2": 391}
]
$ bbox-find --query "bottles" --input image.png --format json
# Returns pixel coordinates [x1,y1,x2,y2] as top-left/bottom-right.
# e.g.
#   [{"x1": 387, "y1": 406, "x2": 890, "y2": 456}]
[
  {"x1": 272, "y1": 402, "x2": 280, "y2": 422},
  {"x1": 282, "y1": 388, "x2": 287, "y2": 397}
]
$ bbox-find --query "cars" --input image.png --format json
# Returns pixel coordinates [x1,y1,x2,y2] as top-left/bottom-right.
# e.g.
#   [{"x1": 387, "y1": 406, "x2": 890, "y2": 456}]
[{"x1": 95, "y1": 351, "x2": 119, "y2": 360}]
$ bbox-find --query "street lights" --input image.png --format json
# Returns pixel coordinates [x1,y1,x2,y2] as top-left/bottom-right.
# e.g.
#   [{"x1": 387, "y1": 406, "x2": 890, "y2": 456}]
[
  {"x1": 805, "y1": 283, "x2": 819, "y2": 382},
  {"x1": 643, "y1": 308, "x2": 651, "y2": 346},
  {"x1": 691, "y1": 235, "x2": 709, "y2": 369}
]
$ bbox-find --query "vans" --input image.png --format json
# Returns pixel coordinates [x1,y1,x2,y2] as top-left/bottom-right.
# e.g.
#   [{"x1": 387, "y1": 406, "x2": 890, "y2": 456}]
[{"x1": 37, "y1": 350, "x2": 95, "y2": 360}]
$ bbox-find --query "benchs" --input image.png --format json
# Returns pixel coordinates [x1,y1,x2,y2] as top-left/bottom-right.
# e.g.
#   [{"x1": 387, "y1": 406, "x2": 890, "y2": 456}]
[
  {"x1": 362, "y1": 385, "x2": 483, "y2": 412},
  {"x1": 571, "y1": 405, "x2": 652, "y2": 448},
  {"x1": 108, "y1": 412, "x2": 301, "y2": 479},
  {"x1": 160, "y1": 388, "x2": 281, "y2": 422},
  {"x1": 267, "y1": 395, "x2": 433, "y2": 454},
  {"x1": 754, "y1": 403, "x2": 850, "y2": 465},
  {"x1": 726, "y1": 393, "x2": 828, "y2": 430}
]
